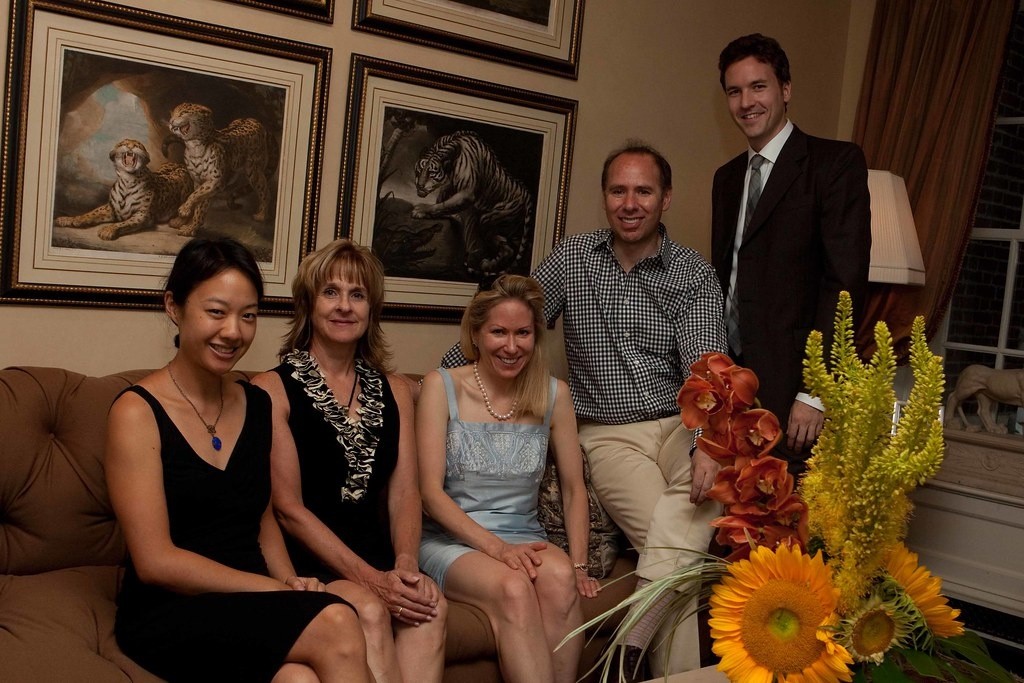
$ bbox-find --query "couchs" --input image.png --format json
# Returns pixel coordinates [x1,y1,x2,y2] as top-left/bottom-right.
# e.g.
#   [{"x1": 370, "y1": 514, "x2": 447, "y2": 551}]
[{"x1": 1, "y1": 362, "x2": 640, "y2": 682}]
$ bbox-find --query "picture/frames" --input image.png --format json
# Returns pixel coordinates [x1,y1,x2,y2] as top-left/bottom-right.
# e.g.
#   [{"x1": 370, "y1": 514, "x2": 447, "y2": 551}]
[
  {"x1": 2, "y1": 1, "x2": 332, "y2": 318},
  {"x1": 351, "y1": 0, "x2": 588, "y2": 83},
  {"x1": 219, "y1": 1, "x2": 337, "y2": 29},
  {"x1": 336, "y1": 53, "x2": 581, "y2": 328}
]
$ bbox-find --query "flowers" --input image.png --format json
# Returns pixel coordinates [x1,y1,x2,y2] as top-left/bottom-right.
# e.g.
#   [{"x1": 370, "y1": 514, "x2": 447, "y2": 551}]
[{"x1": 550, "y1": 292, "x2": 968, "y2": 683}]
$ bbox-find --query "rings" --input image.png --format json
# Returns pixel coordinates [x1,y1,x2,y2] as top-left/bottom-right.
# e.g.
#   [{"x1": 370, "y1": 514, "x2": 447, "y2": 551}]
[
  {"x1": 396, "y1": 605, "x2": 406, "y2": 615},
  {"x1": 586, "y1": 576, "x2": 598, "y2": 582}
]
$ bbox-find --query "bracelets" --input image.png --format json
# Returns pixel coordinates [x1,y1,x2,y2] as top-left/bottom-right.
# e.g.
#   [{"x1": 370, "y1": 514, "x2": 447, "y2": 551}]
[{"x1": 572, "y1": 559, "x2": 591, "y2": 573}]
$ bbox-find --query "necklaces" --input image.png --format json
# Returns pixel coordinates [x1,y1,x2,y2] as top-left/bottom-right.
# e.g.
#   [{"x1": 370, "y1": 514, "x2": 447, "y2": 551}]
[
  {"x1": 472, "y1": 360, "x2": 519, "y2": 421},
  {"x1": 339, "y1": 366, "x2": 362, "y2": 422},
  {"x1": 163, "y1": 358, "x2": 228, "y2": 451}
]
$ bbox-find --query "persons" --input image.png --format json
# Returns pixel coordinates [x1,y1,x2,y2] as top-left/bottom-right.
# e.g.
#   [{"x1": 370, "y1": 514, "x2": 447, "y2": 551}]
[
  {"x1": 98, "y1": 232, "x2": 379, "y2": 683},
  {"x1": 412, "y1": 263, "x2": 602, "y2": 683},
  {"x1": 704, "y1": 33, "x2": 876, "y2": 493},
  {"x1": 246, "y1": 240, "x2": 447, "y2": 683},
  {"x1": 390, "y1": 140, "x2": 730, "y2": 683}
]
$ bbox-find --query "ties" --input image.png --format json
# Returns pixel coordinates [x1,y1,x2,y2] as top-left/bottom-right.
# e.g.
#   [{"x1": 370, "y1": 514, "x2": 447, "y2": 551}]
[{"x1": 742, "y1": 154, "x2": 765, "y2": 240}]
